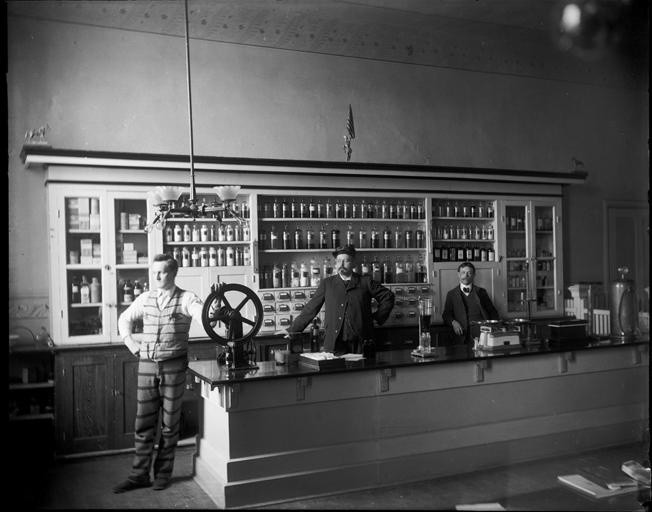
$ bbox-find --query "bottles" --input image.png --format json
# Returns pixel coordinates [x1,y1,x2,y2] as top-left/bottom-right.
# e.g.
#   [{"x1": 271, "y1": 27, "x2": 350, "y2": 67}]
[
  {"x1": 433, "y1": 245, "x2": 495, "y2": 262},
  {"x1": 431, "y1": 223, "x2": 494, "y2": 240},
  {"x1": 257, "y1": 201, "x2": 425, "y2": 219},
  {"x1": 432, "y1": 200, "x2": 493, "y2": 217},
  {"x1": 261, "y1": 258, "x2": 426, "y2": 290},
  {"x1": 503, "y1": 215, "x2": 550, "y2": 231},
  {"x1": 507, "y1": 248, "x2": 552, "y2": 287},
  {"x1": 310, "y1": 320, "x2": 320, "y2": 352},
  {"x1": 69, "y1": 274, "x2": 150, "y2": 304},
  {"x1": 164, "y1": 221, "x2": 250, "y2": 268},
  {"x1": 258, "y1": 224, "x2": 424, "y2": 248}
]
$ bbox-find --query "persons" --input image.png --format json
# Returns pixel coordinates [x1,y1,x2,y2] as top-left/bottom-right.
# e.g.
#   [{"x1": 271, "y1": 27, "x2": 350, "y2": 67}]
[
  {"x1": 273, "y1": 245, "x2": 396, "y2": 357},
  {"x1": 441, "y1": 262, "x2": 499, "y2": 345},
  {"x1": 111, "y1": 255, "x2": 226, "y2": 494}
]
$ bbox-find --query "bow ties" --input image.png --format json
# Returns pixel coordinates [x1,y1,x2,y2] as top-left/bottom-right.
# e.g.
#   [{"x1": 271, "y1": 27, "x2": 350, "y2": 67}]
[{"x1": 462, "y1": 287, "x2": 470, "y2": 293}]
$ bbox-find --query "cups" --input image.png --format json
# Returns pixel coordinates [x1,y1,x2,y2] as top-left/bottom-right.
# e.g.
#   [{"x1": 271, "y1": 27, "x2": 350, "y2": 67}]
[{"x1": 269, "y1": 343, "x2": 289, "y2": 366}]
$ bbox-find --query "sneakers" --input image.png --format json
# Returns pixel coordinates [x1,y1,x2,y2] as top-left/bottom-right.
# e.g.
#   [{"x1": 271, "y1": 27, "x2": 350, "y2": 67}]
[
  {"x1": 152, "y1": 478, "x2": 169, "y2": 490},
  {"x1": 112, "y1": 481, "x2": 151, "y2": 493}
]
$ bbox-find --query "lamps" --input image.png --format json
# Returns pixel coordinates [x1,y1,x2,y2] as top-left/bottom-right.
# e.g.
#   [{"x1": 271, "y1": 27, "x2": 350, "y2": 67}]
[{"x1": 145, "y1": 0, "x2": 249, "y2": 232}]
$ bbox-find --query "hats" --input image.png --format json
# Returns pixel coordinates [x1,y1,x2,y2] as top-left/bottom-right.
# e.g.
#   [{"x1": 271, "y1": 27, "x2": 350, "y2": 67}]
[{"x1": 332, "y1": 245, "x2": 357, "y2": 257}]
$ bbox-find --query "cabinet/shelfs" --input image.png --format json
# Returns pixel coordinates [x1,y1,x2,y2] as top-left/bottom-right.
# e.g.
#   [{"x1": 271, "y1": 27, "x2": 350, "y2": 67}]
[
  {"x1": 55, "y1": 343, "x2": 141, "y2": 462},
  {"x1": 260, "y1": 334, "x2": 315, "y2": 362},
  {"x1": 44, "y1": 165, "x2": 564, "y2": 349},
  {"x1": 9, "y1": 347, "x2": 54, "y2": 420},
  {"x1": 178, "y1": 340, "x2": 260, "y2": 440}
]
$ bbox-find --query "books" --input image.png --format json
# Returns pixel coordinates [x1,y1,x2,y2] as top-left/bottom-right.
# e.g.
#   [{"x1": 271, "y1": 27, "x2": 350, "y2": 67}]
[{"x1": 555, "y1": 455, "x2": 652, "y2": 497}]
